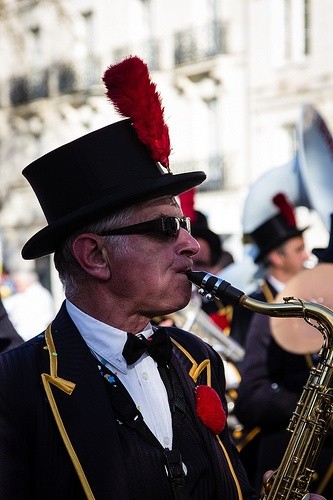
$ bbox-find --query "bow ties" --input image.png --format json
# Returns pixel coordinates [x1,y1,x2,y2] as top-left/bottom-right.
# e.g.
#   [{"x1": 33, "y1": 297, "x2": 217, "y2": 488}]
[{"x1": 122, "y1": 329, "x2": 174, "y2": 367}]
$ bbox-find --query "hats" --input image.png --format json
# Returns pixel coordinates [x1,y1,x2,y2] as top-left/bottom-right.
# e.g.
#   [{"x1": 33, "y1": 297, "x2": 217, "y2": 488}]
[
  {"x1": 20, "y1": 57, "x2": 207, "y2": 261},
  {"x1": 247, "y1": 192, "x2": 311, "y2": 264}
]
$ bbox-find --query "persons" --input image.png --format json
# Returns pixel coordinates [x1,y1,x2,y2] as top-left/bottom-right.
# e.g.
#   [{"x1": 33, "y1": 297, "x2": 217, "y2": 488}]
[
  {"x1": 0, "y1": 294, "x2": 24, "y2": 357},
  {"x1": 233, "y1": 212, "x2": 333, "y2": 500},
  {"x1": 227, "y1": 213, "x2": 312, "y2": 351},
  {"x1": 3, "y1": 270, "x2": 54, "y2": 342},
  {"x1": 158, "y1": 210, "x2": 262, "y2": 453},
  {"x1": 0, "y1": 116, "x2": 326, "y2": 500}
]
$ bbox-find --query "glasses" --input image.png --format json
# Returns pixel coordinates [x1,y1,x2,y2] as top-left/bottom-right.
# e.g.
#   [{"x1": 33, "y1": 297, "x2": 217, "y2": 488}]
[{"x1": 97, "y1": 215, "x2": 192, "y2": 244}]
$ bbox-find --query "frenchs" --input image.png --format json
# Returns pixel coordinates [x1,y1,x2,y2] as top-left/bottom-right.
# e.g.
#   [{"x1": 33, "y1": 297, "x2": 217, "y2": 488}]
[{"x1": 240, "y1": 101, "x2": 333, "y2": 289}]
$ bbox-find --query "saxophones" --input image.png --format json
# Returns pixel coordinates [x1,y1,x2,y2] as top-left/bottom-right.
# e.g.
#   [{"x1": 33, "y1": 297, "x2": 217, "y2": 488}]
[{"x1": 182, "y1": 268, "x2": 333, "y2": 500}]
